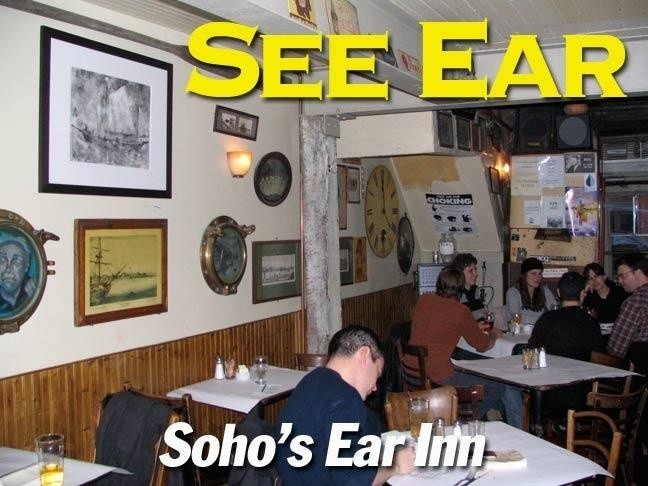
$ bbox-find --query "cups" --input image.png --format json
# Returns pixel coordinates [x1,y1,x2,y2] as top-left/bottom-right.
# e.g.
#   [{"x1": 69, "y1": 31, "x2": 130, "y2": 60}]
[
  {"x1": 511, "y1": 314, "x2": 533, "y2": 337},
  {"x1": 408, "y1": 399, "x2": 431, "y2": 441},
  {"x1": 432, "y1": 251, "x2": 439, "y2": 264},
  {"x1": 34, "y1": 434, "x2": 65, "y2": 486},
  {"x1": 549, "y1": 304, "x2": 560, "y2": 311},
  {"x1": 579, "y1": 305, "x2": 591, "y2": 315},
  {"x1": 254, "y1": 356, "x2": 269, "y2": 386},
  {"x1": 483, "y1": 311, "x2": 495, "y2": 332}
]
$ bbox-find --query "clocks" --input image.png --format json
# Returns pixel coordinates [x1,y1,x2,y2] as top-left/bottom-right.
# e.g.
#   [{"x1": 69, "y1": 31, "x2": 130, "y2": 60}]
[{"x1": 365, "y1": 166, "x2": 399, "y2": 257}]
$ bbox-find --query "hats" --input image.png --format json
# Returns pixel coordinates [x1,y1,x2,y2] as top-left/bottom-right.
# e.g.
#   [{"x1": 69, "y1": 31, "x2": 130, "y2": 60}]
[{"x1": 521, "y1": 257, "x2": 543, "y2": 273}]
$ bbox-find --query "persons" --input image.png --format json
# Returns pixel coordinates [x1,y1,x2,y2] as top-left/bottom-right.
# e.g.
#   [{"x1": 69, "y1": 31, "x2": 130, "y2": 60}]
[
  {"x1": 1, "y1": 239, "x2": 38, "y2": 313},
  {"x1": 274, "y1": 324, "x2": 417, "y2": 486},
  {"x1": 404, "y1": 253, "x2": 648, "y2": 430}
]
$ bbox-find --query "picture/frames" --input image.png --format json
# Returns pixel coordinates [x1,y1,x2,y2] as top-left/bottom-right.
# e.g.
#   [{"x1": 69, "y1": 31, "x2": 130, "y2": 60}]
[
  {"x1": 213, "y1": 105, "x2": 258, "y2": 141},
  {"x1": 253, "y1": 240, "x2": 301, "y2": 303},
  {"x1": 39, "y1": 26, "x2": 173, "y2": 198},
  {"x1": 340, "y1": 237, "x2": 353, "y2": 285},
  {"x1": 74, "y1": 219, "x2": 168, "y2": 327},
  {"x1": 437, "y1": 112, "x2": 454, "y2": 148},
  {"x1": 471, "y1": 121, "x2": 482, "y2": 152},
  {"x1": 489, "y1": 167, "x2": 500, "y2": 193},
  {"x1": 457, "y1": 117, "x2": 471, "y2": 151}
]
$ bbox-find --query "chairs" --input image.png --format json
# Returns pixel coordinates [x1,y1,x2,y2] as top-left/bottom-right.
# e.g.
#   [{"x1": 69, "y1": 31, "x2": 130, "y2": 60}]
[{"x1": 92, "y1": 306, "x2": 648, "y2": 486}]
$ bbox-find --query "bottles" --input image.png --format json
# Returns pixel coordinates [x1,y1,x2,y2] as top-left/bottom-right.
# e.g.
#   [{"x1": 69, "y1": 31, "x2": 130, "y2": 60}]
[
  {"x1": 433, "y1": 417, "x2": 484, "y2": 449},
  {"x1": 522, "y1": 348, "x2": 548, "y2": 371},
  {"x1": 215, "y1": 356, "x2": 236, "y2": 380}
]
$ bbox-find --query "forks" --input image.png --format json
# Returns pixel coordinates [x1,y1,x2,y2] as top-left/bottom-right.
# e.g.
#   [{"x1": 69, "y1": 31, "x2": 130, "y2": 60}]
[{"x1": 454, "y1": 470, "x2": 475, "y2": 486}]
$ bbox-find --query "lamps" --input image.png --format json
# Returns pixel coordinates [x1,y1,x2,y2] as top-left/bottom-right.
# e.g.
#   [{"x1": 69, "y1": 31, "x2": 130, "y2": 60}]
[{"x1": 227, "y1": 152, "x2": 252, "y2": 178}]
